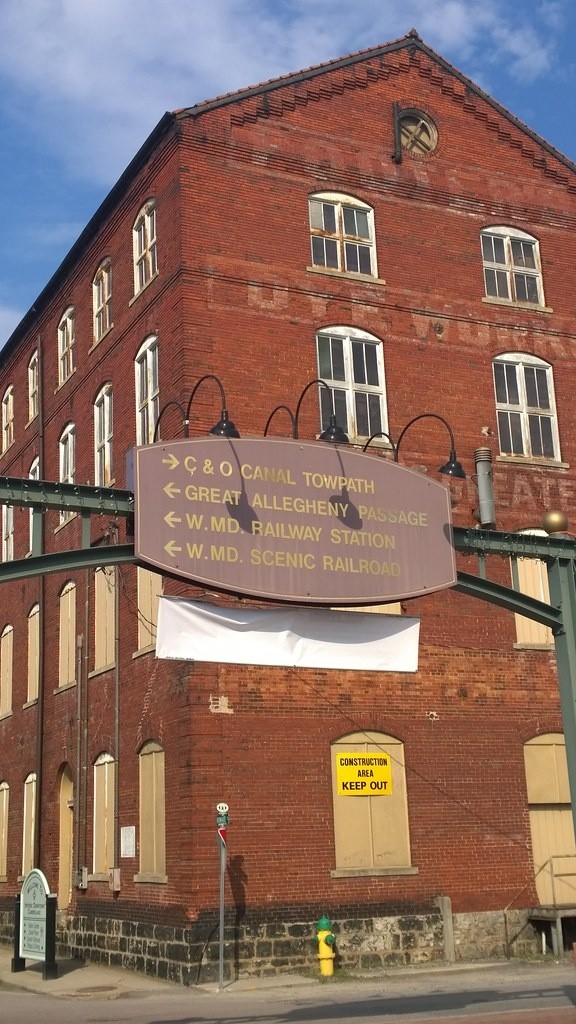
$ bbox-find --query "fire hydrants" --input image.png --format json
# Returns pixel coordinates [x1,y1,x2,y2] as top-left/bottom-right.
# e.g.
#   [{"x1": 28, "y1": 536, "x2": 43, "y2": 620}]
[{"x1": 313, "y1": 913, "x2": 336, "y2": 977}]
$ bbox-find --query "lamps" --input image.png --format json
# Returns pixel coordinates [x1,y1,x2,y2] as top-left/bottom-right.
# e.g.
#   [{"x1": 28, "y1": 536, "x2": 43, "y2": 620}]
[
  {"x1": 154, "y1": 375, "x2": 240, "y2": 438},
  {"x1": 475, "y1": 447, "x2": 496, "y2": 525},
  {"x1": 264, "y1": 380, "x2": 350, "y2": 443},
  {"x1": 363, "y1": 414, "x2": 466, "y2": 479}
]
